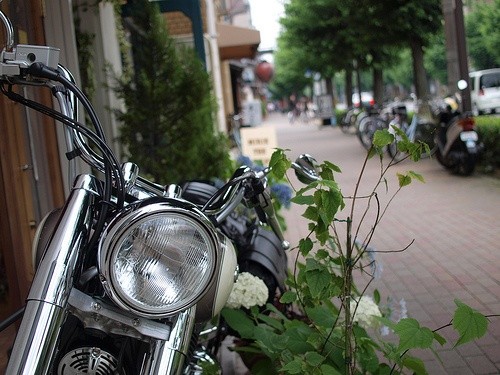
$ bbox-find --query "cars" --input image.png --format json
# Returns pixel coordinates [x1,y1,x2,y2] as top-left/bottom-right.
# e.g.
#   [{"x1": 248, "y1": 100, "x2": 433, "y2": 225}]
[{"x1": 352, "y1": 92, "x2": 373, "y2": 108}]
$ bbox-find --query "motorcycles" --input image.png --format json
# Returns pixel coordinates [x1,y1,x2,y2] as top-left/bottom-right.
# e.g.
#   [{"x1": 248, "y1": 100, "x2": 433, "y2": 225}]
[{"x1": 0, "y1": 12, "x2": 323, "y2": 375}]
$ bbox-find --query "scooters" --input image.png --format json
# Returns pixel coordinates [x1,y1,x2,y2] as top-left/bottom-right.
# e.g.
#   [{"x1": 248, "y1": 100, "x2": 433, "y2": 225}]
[{"x1": 414, "y1": 79, "x2": 485, "y2": 177}]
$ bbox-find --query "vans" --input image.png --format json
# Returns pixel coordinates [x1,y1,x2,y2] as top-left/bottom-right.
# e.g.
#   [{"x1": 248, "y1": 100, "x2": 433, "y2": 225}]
[{"x1": 470, "y1": 68, "x2": 500, "y2": 115}]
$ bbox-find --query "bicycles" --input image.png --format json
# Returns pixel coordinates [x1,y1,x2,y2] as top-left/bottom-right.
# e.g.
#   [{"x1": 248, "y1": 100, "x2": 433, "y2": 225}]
[{"x1": 339, "y1": 92, "x2": 419, "y2": 162}]
